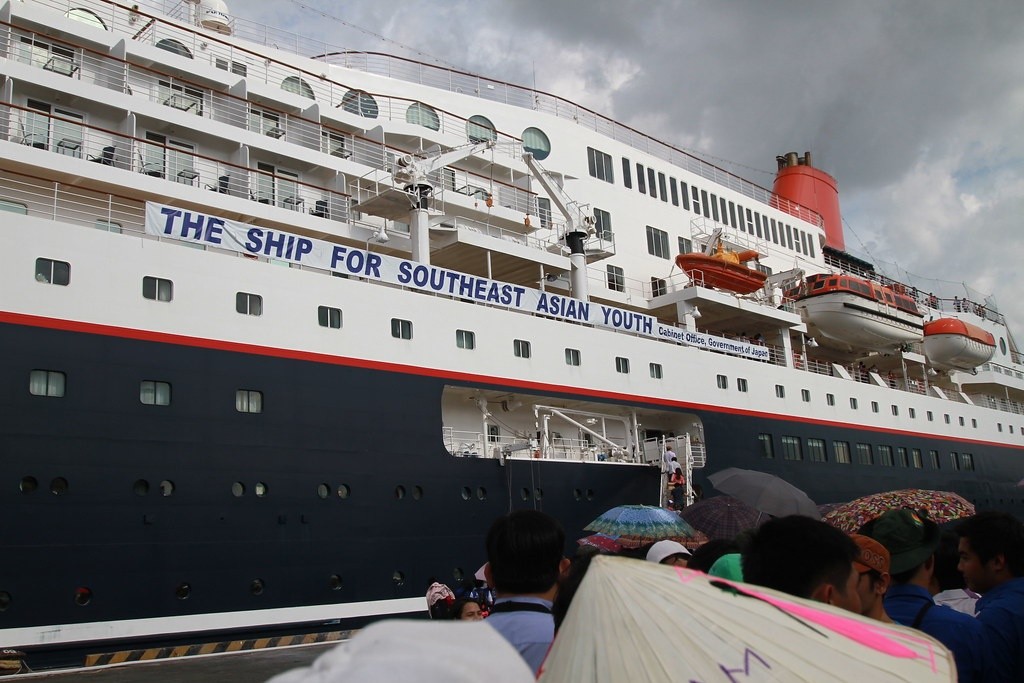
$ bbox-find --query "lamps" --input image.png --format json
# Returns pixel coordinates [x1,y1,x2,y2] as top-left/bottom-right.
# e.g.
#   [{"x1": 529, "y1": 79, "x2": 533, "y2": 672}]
[
  {"x1": 682, "y1": 306, "x2": 701, "y2": 326},
  {"x1": 367, "y1": 226, "x2": 389, "y2": 265},
  {"x1": 804, "y1": 337, "x2": 819, "y2": 348}
]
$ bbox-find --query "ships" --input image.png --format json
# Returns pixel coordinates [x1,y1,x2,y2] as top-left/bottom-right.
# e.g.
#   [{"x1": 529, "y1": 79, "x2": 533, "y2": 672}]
[{"x1": 0, "y1": 1, "x2": 1024, "y2": 671}]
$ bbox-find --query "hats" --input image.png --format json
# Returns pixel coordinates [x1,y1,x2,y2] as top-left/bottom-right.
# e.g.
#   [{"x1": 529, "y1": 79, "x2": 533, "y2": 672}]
[
  {"x1": 848, "y1": 534, "x2": 889, "y2": 574},
  {"x1": 646, "y1": 540, "x2": 692, "y2": 564},
  {"x1": 858, "y1": 509, "x2": 941, "y2": 574}
]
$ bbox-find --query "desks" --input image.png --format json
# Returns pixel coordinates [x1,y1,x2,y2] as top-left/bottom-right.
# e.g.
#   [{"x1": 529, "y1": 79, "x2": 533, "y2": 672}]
[
  {"x1": 177, "y1": 169, "x2": 201, "y2": 188},
  {"x1": 267, "y1": 126, "x2": 286, "y2": 139},
  {"x1": 56, "y1": 137, "x2": 82, "y2": 158},
  {"x1": 331, "y1": 147, "x2": 353, "y2": 161},
  {"x1": 283, "y1": 195, "x2": 305, "y2": 212},
  {"x1": 163, "y1": 93, "x2": 198, "y2": 112},
  {"x1": 42, "y1": 57, "x2": 81, "y2": 80}
]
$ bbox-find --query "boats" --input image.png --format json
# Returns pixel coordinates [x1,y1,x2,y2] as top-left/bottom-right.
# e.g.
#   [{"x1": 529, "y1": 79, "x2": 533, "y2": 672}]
[
  {"x1": 923, "y1": 318, "x2": 997, "y2": 371},
  {"x1": 782, "y1": 272, "x2": 923, "y2": 356},
  {"x1": 675, "y1": 249, "x2": 767, "y2": 295}
]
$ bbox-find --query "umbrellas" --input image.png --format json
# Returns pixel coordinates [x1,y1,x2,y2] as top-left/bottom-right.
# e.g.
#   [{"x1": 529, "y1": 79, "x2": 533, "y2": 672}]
[
  {"x1": 615, "y1": 528, "x2": 709, "y2": 550},
  {"x1": 708, "y1": 467, "x2": 822, "y2": 521},
  {"x1": 816, "y1": 503, "x2": 844, "y2": 519},
  {"x1": 574, "y1": 530, "x2": 622, "y2": 554},
  {"x1": 580, "y1": 503, "x2": 696, "y2": 538},
  {"x1": 677, "y1": 495, "x2": 768, "y2": 540},
  {"x1": 823, "y1": 486, "x2": 978, "y2": 533}
]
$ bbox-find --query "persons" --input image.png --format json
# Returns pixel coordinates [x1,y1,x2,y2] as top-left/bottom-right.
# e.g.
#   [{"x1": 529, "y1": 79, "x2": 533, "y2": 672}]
[
  {"x1": 909, "y1": 287, "x2": 986, "y2": 319},
  {"x1": 729, "y1": 331, "x2": 767, "y2": 362},
  {"x1": 258, "y1": 497, "x2": 1024, "y2": 682},
  {"x1": 663, "y1": 446, "x2": 690, "y2": 515},
  {"x1": 858, "y1": 362, "x2": 896, "y2": 389}
]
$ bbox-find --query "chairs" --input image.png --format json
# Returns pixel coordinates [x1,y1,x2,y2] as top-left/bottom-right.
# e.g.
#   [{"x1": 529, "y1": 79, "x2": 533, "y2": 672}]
[
  {"x1": 137, "y1": 149, "x2": 164, "y2": 178},
  {"x1": 250, "y1": 189, "x2": 270, "y2": 204},
  {"x1": 86, "y1": 144, "x2": 117, "y2": 167},
  {"x1": 18, "y1": 118, "x2": 48, "y2": 151},
  {"x1": 309, "y1": 201, "x2": 331, "y2": 220},
  {"x1": 204, "y1": 175, "x2": 231, "y2": 196}
]
